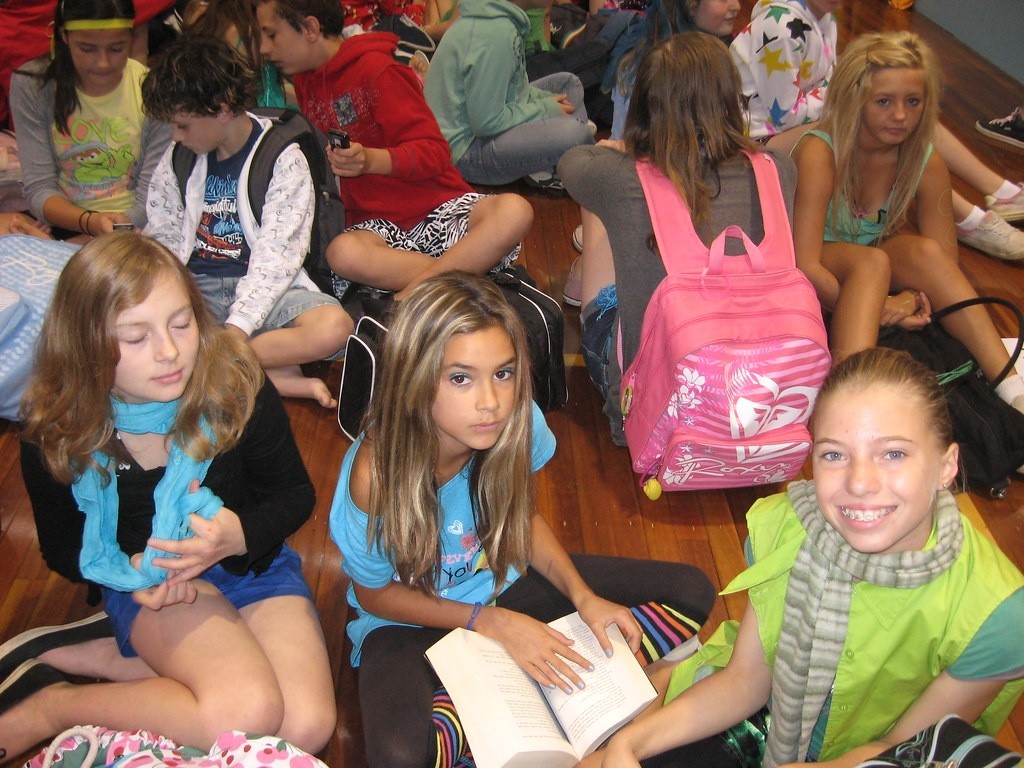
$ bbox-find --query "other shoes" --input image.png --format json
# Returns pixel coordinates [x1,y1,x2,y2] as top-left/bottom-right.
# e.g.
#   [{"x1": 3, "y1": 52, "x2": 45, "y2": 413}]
[
  {"x1": 564, "y1": 256, "x2": 584, "y2": 307},
  {"x1": 1, "y1": 611, "x2": 116, "y2": 682},
  {"x1": 524, "y1": 171, "x2": 565, "y2": 189},
  {"x1": 0, "y1": 658, "x2": 65, "y2": 718},
  {"x1": 573, "y1": 224, "x2": 583, "y2": 252}
]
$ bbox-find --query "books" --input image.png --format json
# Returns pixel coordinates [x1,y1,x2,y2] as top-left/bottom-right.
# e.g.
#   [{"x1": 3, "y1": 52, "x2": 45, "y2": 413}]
[{"x1": 424, "y1": 612, "x2": 660, "y2": 768}]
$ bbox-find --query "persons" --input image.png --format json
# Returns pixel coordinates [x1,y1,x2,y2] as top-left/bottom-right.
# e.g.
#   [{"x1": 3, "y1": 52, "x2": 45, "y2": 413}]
[
  {"x1": 788, "y1": 29, "x2": 1024, "y2": 413},
  {"x1": 4, "y1": 0, "x2": 1024, "y2": 305},
  {"x1": 558, "y1": 33, "x2": 798, "y2": 447},
  {"x1": 0, "y1": 232, "x2": 338, "y2": 764},
  {"x1": 573, "y1": 347, "x2": 1024, "y2": 768},
  {"x1": 0, "y1": 0, "x2": 171, "y2": 249},
  {"x1": 142, "y1": 38, "x2": 354, "y2": 407},
  {"x1": 330, "y1": 269, "x2": 716, "y2": 768}
]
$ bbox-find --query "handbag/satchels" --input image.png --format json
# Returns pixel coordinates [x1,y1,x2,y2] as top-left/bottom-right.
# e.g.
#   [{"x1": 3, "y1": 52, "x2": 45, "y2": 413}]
[
  {"x1": 854, "y1": 714, "x2": 1022, "y2": 767},
  {"x1": 338, "y1": 267, "x2": 569, "y2": 443},
  {"x1": 877, "y1": 296, "x2": 1024, "y2": 498}
]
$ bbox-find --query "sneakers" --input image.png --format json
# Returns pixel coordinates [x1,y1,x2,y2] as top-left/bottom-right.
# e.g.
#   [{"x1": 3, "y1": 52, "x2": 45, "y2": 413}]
[
  {"x1": 975, "y1": 107, "x2": 1024, "y2": 148},
  {"x1": 988, "y1": 182, "x2": 1024, "y2": 221},
  {"x1": 956, "y1": 210, "x2": 1024, "y2": 261}
]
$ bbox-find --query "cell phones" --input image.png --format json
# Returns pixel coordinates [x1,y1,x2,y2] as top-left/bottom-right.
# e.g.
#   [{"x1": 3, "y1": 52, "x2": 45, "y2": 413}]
[
  {"x1": 328, "y1": 127, "x2": 350, "y2": 153},
  {"x1": 113, "y1": 223, "x2": 135, "y2": 231}
]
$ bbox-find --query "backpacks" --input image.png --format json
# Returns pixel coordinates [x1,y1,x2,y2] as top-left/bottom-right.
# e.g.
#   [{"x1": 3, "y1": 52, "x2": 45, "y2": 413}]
[
  {"x1": 525, "y1": 9, "x2": 647, "y2": 128},
  {"x1": 617, "y1": 150, "x2": 831, "y2": 499},
  {"x1": 171, "y1": 105, "x2": 344, "y2": 302}
]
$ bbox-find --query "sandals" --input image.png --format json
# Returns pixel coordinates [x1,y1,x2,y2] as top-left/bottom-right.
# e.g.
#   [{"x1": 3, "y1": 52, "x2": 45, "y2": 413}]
[{"x1": 409, "y1": 50, "x2": 429, "y2": 86}]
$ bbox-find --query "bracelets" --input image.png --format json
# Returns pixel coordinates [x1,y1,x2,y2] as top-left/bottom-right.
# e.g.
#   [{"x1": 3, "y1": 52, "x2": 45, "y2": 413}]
[
  {"x1": 466, "y1": 601, "x2": 481, "y2": 630},
  {"x1": 79, "y1": 210, "x2": 99, "y2": 237}
]
